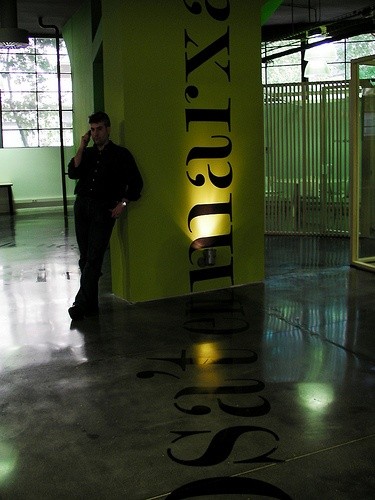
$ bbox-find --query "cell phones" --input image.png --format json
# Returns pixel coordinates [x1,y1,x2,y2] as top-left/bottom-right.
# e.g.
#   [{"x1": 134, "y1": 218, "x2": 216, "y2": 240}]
[{"x1": 83, "y1": 130, "x2": 91, "y2": 143}]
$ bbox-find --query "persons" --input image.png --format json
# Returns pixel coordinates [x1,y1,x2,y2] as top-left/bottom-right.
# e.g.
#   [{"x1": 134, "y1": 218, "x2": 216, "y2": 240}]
[{"x1": 67, "y1": 111, "x2": 142, "y2": 319}]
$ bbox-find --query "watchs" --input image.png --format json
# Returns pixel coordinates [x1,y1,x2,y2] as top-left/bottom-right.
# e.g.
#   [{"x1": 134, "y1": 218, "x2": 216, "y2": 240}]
[{"x1": 120, "y1": 200, "x2": 127, "y2": 206}]
